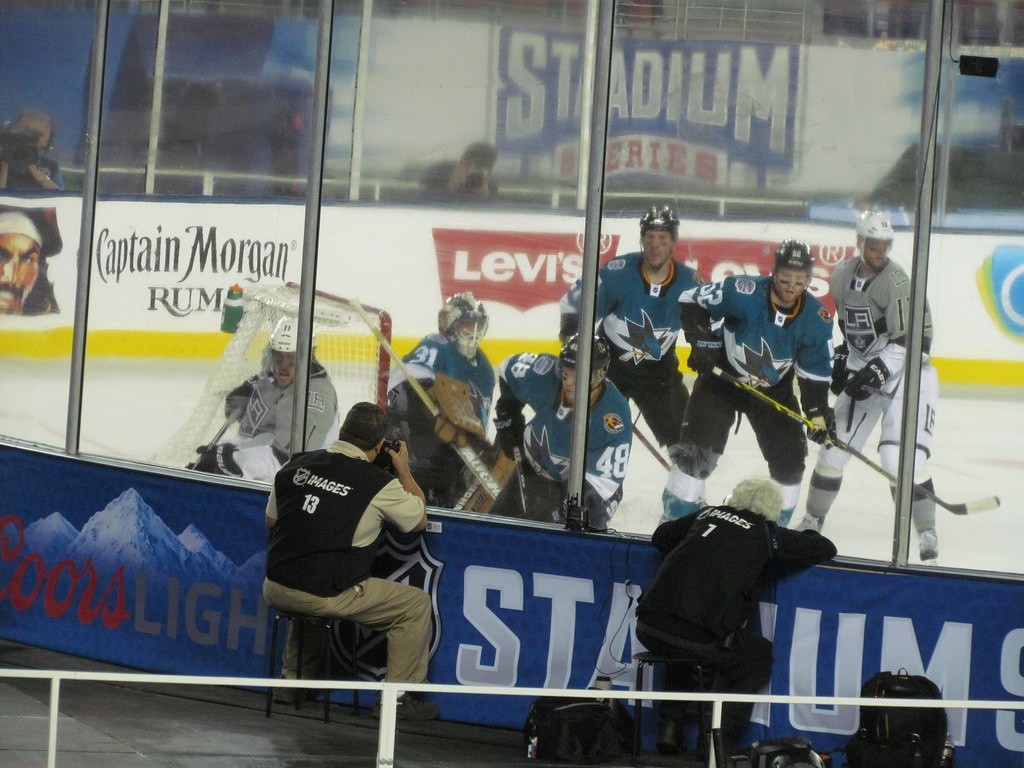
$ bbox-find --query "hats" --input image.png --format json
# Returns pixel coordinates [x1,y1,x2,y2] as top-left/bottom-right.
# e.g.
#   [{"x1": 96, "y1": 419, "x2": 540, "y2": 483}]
[{"x1": 467, "y1": 142, "x2": 496, "y2": 168}]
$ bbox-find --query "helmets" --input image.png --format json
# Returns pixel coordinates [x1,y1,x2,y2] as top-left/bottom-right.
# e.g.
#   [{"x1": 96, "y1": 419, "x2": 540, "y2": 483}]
[
  {"x1": 269, "y1": 316, "x2": 316, "y2": 352},
  {"x1": 436, "y1": 292, "x2": 488, "y2": 357},
  {"x1": 776, "y1": 238, "x2": 816, "y2": 269},
  {"x1": 559, "y1": 333, "x2": 612, "y2": 369},
  {"x1": 640, "y1": 206, "x2": 679, "y2": 240},
  {"x1": 857, "y1": 209, "x2": 895, "y2": 242}
]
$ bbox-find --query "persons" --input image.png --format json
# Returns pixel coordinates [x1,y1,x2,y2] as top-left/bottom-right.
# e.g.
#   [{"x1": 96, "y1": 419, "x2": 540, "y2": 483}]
[
  {"x1": 185, "y1": 316, "x2": 338, "y2": 481},
  {"x1": 493, "y1": 334, "x2": 632, "y2": 532},
  {"x1": 558, "y1": 204, "x2": 711, "y2": 465},
  {"x1": 635, "y1": 476, "x2": 838, "y2": 761},
  {"x1": 264, "y1": 402, "x2": 439, "y2": 722},
  {"x1": 662, "y1": 239, "x2": 834, "y2": 525},
  {"x1": 616, "y1": 0, "x2": 663, "y2": 26},
  {"x1": 0, "y1": 106, "x2": 66, "y2": 189},
  {"x1": 416, "y1": 141, "x2": 498, "y2": 203},
  {"x1": 387, "y1": 293, "x2": 495, "y2": 507}
]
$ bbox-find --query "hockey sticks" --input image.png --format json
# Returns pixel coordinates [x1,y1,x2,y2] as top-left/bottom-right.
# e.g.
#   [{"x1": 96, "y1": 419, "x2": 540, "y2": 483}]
[
  {"x1": 190, "y1": 408, "x2": 243, "y2": 471},
  {"x1": 350, "y1": 296, "x2": 502, "y2": 501},
  {"x1": 861, "y1": 373, "x2": 902, "y2": 401},
  {"x1": 512, "y1": 446, "x2": 529, "y2": 515},
  {"x1": 712, "y1": 366, "x2": 1003, "y2": 517},
  {"x1": 631, "y1": 420, "x2": 711, "y2": 510}
]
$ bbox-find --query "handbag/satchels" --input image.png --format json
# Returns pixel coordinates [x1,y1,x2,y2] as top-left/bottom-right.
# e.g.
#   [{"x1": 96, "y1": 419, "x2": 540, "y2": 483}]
[{"x1": 524, "y1": 687, "x2": 642, "y2": 763}]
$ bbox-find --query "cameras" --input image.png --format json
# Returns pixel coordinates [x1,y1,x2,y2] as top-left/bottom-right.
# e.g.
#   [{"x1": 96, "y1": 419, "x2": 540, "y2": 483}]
[{"x1": 372, "y1": 440, "x2": 398, "y2": 474}]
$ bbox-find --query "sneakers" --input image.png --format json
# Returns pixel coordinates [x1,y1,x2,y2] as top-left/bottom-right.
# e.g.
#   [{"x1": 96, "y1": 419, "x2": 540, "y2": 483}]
[
  {"x1": 272, "y1": 676, "x2": 307, "y2": 704},
  {"x1": 795, "y1": 514, "x2": 824, "y2": 534},
  {"x1": 372, "y1": 692, "x2": 439, "y2": 721},
  {"x1": 918, "y1": 528, "x2": 938, "y2": 565}
]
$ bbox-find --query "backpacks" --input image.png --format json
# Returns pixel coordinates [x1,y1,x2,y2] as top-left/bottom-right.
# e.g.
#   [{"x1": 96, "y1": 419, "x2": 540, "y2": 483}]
[{"x1": 845, "y1": 667, "x2": 947, "y2": 768}]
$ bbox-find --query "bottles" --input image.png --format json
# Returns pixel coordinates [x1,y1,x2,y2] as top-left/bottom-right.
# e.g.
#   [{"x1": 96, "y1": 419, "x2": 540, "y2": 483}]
[
  {"x1": 220, "y1": 283, "x2": 244, "y2": 333},
  {"x1": 941, "y1": 731, "x2": 954, "y2": 759},
  {"x1": 596, "y1": 676, "x2": 614, "y2": 709}
]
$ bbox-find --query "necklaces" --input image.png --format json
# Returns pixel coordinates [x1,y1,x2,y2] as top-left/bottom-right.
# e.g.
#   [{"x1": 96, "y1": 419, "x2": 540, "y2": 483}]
[{"x1": 793, "y1": 209, "x2": 939, "y2": 561}]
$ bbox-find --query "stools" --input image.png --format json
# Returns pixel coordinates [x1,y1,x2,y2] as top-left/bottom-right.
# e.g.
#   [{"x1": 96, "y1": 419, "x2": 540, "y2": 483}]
[
  {"x1": 265, "y1": 609, "x2": 359, "y2": 725},
  {"x1": 631, "y1": 651, "x2": 712, "y2": 764}
]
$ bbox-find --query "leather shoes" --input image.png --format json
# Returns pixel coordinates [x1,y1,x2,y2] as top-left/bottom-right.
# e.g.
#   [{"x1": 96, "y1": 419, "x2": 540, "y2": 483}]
[
  {"x1": 697, "y1": 732, "x2": 741, "y2": 756},
  {"x1": 657, "y1": 716, "x2": 687, "y2": 754}
]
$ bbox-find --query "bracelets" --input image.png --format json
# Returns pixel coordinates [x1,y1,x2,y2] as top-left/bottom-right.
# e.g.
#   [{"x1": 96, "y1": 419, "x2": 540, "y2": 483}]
[{"x1": 38, "y1": 175, "x2": 49, "y2": 184}]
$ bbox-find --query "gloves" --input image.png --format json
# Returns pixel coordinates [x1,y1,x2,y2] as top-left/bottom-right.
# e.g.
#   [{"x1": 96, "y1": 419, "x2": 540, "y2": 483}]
[
  {"x1": 547, "y1": 482, "x2": 601, "y2": 525},
  {"x1": 687, "y1": 339, "x2": 724, "y2": 380},
  {"x1": 224, "y1": 375, "x2": 259, "y2": 423},
  {"x1": 185, "y1": 443, "x2": 243, "y2": 478},
  {"x1": 831, "y1": 345, "x2": 850, "y2": 396},
  {"x1": 805, "y1": 404, "x2": 836, "y2": 448},
  {"x1": 493, "y1": 400, "x2": 526, "y2": 460},
  {"x1": 422, "y1": 386, "x2": 466, "y2": 447},
  {"x1": 844, "y1": 358, "x2": 890, "y2": 400}
]
